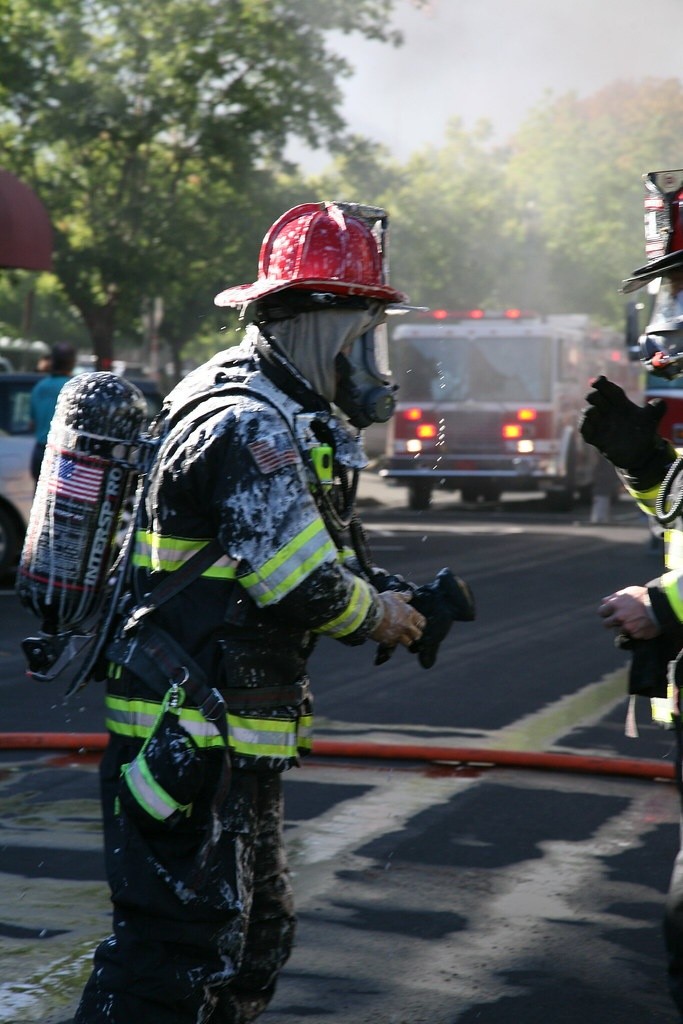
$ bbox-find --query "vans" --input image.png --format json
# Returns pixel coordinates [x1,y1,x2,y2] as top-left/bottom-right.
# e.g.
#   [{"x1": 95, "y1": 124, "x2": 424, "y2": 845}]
[{"x1": 0, "y1": 371, "x2": 167, "y2": 596}]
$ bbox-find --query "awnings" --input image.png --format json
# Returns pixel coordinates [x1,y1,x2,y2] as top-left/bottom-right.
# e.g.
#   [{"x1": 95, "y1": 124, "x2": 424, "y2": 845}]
[{"x1": 0, "y1": 168, "x2": 53, "y2": 270}]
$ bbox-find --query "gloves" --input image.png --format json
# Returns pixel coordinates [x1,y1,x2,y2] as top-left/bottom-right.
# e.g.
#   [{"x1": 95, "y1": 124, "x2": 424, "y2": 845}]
[
  {"x1": 613, "y1": 625, "x2": 681, "y2": 700},
  {"x1": 577, "y1": 375, "x2": 667, "y2": 470},
  {"x1": 373, "y1": 567, "x2": 473, "y2": 671}
]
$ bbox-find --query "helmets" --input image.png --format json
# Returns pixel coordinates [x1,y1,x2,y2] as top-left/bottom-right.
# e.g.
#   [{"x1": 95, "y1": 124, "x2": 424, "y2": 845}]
[{"x1": 214, "y1": 202, "x2": 406, "y2": 307}]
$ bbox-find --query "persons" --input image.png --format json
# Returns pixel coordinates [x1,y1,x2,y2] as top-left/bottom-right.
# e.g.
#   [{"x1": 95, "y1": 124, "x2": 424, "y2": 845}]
[
  {"x1": 579, "y1": 169, "x2": 683, "y2": 1024},
  {"x1": 29, "y1": 346, "x2": 83, "y2": 500},
  {"x1": 58, "y1": 202, "x2": 427, "y2": 1024}
]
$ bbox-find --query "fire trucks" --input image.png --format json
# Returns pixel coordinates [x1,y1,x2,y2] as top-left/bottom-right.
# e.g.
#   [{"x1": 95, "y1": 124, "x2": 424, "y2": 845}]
[
  {"x1": 647, "y1": 356, "x2": 682, "y2": 451},
  {"x1": 379, "y1": 309, "x2": 649, "y2": 518}
]
